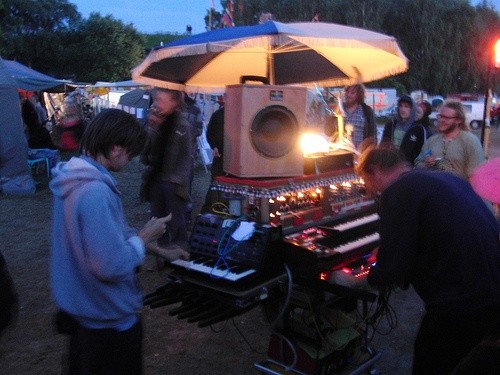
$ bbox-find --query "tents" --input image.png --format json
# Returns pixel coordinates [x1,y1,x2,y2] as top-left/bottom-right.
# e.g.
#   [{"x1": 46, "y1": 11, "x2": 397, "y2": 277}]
[{"x1": 0, "y1": 58, "x2": 225, "y2": 193}]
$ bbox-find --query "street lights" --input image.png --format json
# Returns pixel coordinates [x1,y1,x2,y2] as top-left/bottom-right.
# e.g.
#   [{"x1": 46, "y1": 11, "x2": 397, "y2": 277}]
[{"x1": 478, "y1": 33, "x2": 500, "y2": 157}]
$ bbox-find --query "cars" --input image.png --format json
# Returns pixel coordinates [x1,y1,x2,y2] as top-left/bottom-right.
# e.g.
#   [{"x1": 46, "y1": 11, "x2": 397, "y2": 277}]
[{"x1": 376, "y1": 93, "x2": 500, "y2": 130}]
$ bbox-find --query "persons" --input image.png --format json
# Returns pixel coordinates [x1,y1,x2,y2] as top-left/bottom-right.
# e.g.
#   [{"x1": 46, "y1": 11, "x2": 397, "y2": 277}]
[
  {"x1": 28, "y1": 119, "x2": 65, "y2": 151},
  {"x1": 143, "y1": 87, "x2": 225, "y2": 248},
  {"x1": 327, "y1": 148, "x2": 500, "y2": 375},
  {"x1": 333, "y1": 83, "x2": 486, "y2": 180},
  {"x1": 48, "y1": 108, "x2": 190, "y2": 375},
  {"x1": 81, "y1": 102, "x2": 96, "y2": 122}
]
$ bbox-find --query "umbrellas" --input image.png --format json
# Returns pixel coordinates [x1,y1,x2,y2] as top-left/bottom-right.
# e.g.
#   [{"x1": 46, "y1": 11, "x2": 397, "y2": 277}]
[
  {"x1": 131, "y1": 19, "x2": 411, "y2": 94},
  {"x1": 118, "y1": 89, "x2": 153, "y2": 108}
]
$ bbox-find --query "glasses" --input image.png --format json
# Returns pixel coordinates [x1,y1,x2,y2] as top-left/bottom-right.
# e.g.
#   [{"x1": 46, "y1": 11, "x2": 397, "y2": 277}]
[{"x1": 436, "y1": 113, "x2": 457, "y2": 120}]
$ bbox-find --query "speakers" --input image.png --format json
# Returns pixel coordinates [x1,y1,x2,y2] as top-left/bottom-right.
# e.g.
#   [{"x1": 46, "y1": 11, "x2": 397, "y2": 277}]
[{"x1": 223, "y1": 85, "x2": 307, "y2": 179}]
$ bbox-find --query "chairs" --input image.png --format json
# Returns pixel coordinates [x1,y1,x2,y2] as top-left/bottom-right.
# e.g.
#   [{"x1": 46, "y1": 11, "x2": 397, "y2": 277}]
[{"x1": 27, "y1": 157, "x2": 50, "y2": 182}]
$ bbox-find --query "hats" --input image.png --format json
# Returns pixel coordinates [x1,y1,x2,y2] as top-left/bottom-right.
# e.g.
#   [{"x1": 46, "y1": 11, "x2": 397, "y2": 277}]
[{"x1": 416, "y1": 99, "x2": 432, "y2": 114}]
